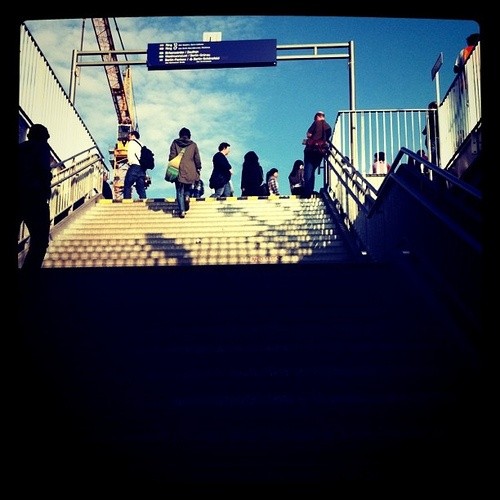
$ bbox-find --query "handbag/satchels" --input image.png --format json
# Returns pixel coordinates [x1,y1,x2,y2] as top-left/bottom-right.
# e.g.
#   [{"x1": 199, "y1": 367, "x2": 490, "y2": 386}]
[
  {"x1": 304, "y1": 139, "x2": 330, "y2": 155},
  {"x1": 165, "y1": 159, "x2": 180, "y2": 184}
]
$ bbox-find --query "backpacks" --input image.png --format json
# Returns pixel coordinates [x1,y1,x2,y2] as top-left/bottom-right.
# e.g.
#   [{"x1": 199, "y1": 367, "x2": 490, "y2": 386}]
[{"x1": 132, "y1": 138, "x2": 154, "y2": 171}]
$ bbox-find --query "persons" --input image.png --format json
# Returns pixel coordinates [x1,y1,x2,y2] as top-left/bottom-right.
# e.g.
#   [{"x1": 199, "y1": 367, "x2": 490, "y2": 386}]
[
  {"x1": 422, "y1": 101, "x2": 440, "y2": 165},
  {"x1": 453, "y1": 34, "x2": 477, "y2": 74},
  {"x1": 341, "y1": 156, "x2": 351, "y2": 171},
  {"x1": 102, "y1": 171, "x2": 113, "y2": 200},
  {"x1": 169, "y1": 128, "x2": 202, "y2": 217},
  {"x1": 265, "y1": 168, "x2": 281, "y2": 196},
  {"x1": 190, "y1": 174, "x2": 205, "y2": 201},
  {"x1": 209, "y1": 142, "x2": 234, "y2": 198},
  {"x1": 14, "y1": 124, "x2": 53, "y2": 273},
  {"x1": 237, "y1": 152, "x2": 268, "y2": 200},
  {"x1": 289, "y1": 161, "x2": 304, "y2": 195},
  {"x1": 301, "y1": 110, "x2": 332, "y2": 199},
  {"x1": 415, "y1": 149, "x2": 428, "y2": 166},
  {"x1": 112, "y1": 132, "x2": 150, "y2": 203},
  {"x1": 370, "y1": 153, "x2": 390, "y2": 175}
]
